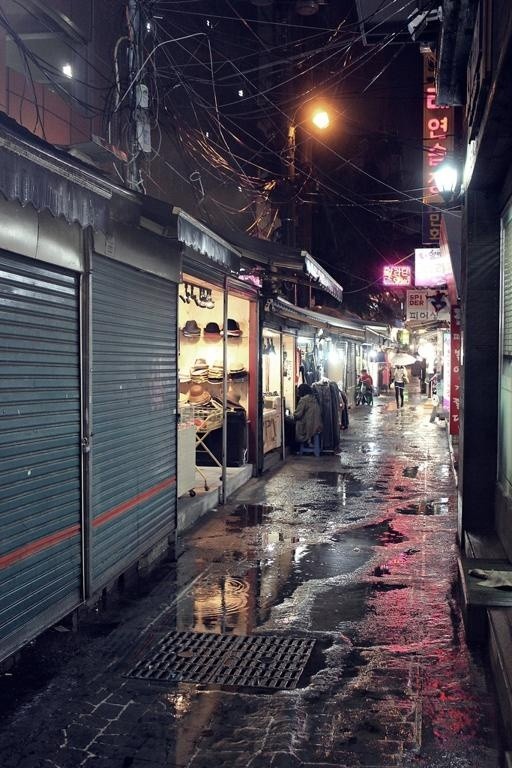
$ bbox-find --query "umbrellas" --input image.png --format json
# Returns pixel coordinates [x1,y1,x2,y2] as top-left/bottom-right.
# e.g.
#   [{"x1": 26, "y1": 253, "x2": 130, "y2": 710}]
[{"x1": 392, "y1": 353, "x2": 416, "y2": 366}]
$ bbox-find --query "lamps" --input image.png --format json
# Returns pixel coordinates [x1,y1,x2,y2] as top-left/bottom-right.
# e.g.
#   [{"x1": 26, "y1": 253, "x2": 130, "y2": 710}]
[{"x1": 435, "y1": 134, "x2": 464, "y2": 203}]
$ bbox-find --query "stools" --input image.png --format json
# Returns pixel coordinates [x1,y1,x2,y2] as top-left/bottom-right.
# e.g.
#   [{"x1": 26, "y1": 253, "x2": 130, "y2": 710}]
[{"x1": 298, "y1": 432, "x2": 322, "y2": 457}]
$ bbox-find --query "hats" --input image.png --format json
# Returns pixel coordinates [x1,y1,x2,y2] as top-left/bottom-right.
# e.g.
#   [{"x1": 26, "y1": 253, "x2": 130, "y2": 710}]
[
  {"x1": 182, "y1": 318, "x2": 241, "y2": 338},
  {"x1": 190, "y1": 358, "x2": 249, "y2": 385},
  {"x1": 185, "y1": 385, "x2": 242, "y2": 410}
]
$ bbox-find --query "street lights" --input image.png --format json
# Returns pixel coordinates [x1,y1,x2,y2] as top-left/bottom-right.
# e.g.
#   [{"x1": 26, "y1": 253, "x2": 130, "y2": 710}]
[{"x1": 287, "y1": 109, "x2": 329, "y2": 306}]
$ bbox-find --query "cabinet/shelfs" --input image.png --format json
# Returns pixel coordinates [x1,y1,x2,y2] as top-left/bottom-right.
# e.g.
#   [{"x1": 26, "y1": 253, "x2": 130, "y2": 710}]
[
  {"x1": 195, "y1": 411, "x2": 246, "y2": 467},
  {"x1": 263, "y1": 407, "x2": 281, "y2": 454},
  {"x1": 177, "y1": 425, "x2": 197, "y2": 499}
]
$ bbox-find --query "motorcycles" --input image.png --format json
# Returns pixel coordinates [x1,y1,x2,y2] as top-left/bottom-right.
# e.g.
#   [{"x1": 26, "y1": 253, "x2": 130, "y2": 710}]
[{"x1": 355, "y1": 374, "x2": 371, "y2": 406}]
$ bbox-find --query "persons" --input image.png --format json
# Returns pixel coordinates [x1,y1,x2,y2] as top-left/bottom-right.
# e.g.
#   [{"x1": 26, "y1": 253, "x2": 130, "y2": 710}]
[
  {"x1": 284, "y1": 382, "x2": 326, "y2": 456},
  {"x1": 393, "y1": 365, "x2": 409, "y2": 408},
  {"x1": 358, "y1": 369, "x2": 374, "y2": 405}
]
